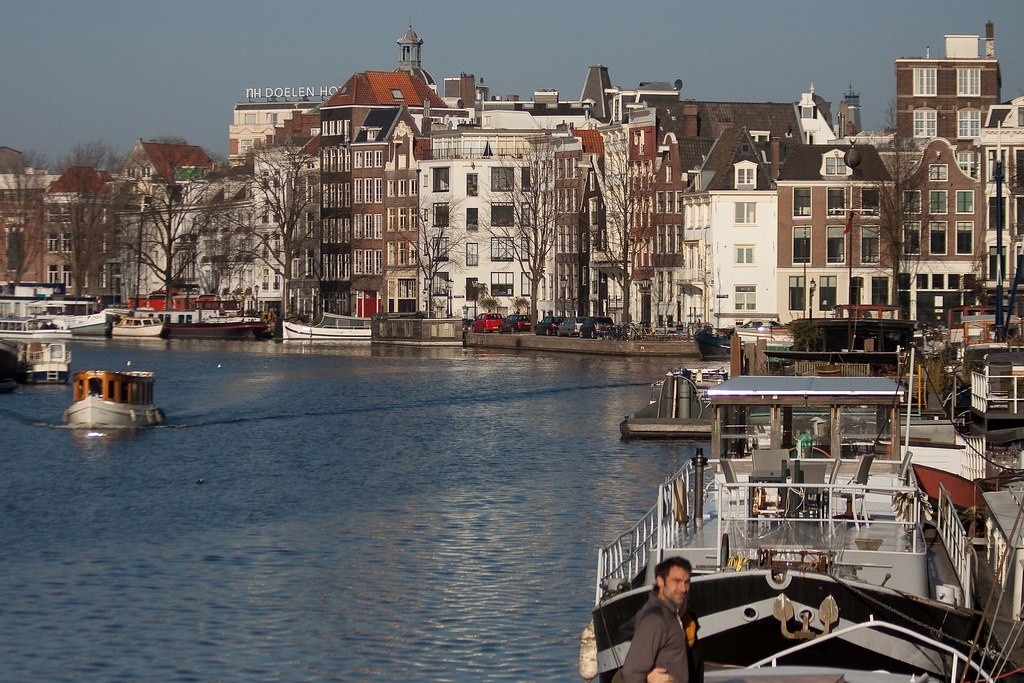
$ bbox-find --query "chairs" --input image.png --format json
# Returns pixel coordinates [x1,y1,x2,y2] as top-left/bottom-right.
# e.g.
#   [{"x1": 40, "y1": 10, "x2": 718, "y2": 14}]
[
  {"x1": 752, "y1": 449, "x2": 792, "y2": 516},
  {"x1": 789, "y1": 459, "x2": 842, "y2": 536},
  {"x1": 718, "y1": 459, "x2": 770, "y2": 533},
  {"x1": 859, "y1": 451, "x2": 912, "y2": 521},
  {"x1": 825, "y1": 453, "x2": 875, "y2": 531}
]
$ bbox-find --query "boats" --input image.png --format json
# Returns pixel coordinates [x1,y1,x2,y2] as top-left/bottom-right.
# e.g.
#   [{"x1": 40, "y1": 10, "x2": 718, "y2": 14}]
[
  {"x1": 21, "y1": 339, "x2": 72, "y2": 386},
  {"x1": 281, "y1": 312, "x2": 371, "y2": 341},
  {"x1": 0, "y1": 289, "x2": 275, "y2": 346},
  {"x1": 60, "y1": 367, "x2": 167, "y2": 432}
]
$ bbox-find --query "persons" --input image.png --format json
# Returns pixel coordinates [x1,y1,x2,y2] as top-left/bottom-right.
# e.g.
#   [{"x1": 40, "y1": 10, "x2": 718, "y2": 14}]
[
  {"x1": 610, "y1": 556, "x2": 705, "y2": 683},
  {"x1": 29, "y1": 321, "x2": 57, "y2": 330}
]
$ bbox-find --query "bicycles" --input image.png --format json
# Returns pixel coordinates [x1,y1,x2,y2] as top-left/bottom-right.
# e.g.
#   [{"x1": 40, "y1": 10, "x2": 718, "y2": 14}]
[{"x1": 602, "y1": 319, "x2": 702, "y2": 342}]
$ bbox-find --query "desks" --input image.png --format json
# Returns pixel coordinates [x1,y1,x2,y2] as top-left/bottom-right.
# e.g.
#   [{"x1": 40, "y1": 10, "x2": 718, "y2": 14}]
[{"x1": 748, "y1": 470, "x2": 781, "y2": 537}]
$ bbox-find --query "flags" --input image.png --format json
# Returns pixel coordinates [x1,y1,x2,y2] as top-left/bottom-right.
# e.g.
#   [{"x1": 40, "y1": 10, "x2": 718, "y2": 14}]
[{"x1": 842, "y1": 211, "x2": 856, "y2": 234}]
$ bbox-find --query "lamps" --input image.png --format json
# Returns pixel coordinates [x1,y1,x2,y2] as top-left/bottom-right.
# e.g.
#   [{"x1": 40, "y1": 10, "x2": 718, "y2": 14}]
[{"x1": 470, "y1": 162, "x2": 474, "y2": 166}]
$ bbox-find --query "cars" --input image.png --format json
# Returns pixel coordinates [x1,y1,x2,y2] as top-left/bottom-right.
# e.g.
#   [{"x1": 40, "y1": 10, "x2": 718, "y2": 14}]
[
  {"x1": 556, "y1": 317, "x2": 588, "y2": 337},
  {"x1": 471, "y1": 312, "x2": 503, "y2": 334},
  {"x1": 535, "y1": 316, "x2": 567, "y2": 336},
  {"x1": 498, "y1": 313, "x2": 531, "y2": 334},
  {"x1": 578, "y1": 316, "x2": 614, "y2": 339}
]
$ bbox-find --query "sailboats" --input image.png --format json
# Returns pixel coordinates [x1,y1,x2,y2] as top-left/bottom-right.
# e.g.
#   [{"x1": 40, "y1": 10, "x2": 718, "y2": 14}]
[{"x1": 578, "y1": 120, "x2": 1024, "y2": 683}]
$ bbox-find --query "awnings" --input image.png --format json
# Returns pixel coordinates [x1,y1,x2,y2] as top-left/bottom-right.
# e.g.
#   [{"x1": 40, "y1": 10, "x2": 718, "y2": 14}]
[{"x1": 680, "y1": 284, "x2": 703, "y2": 296}]
